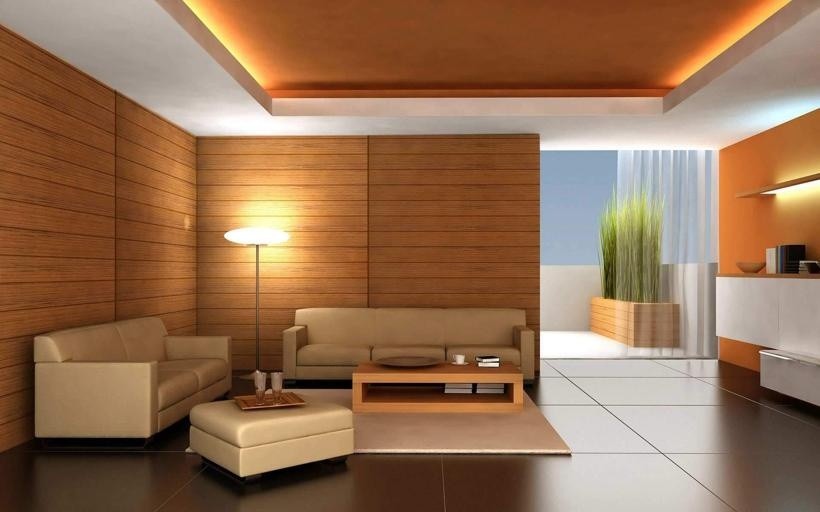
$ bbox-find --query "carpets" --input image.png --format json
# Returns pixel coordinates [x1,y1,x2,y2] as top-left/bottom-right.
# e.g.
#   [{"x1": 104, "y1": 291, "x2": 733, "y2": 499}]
[{"x1": 266, "y1": 388, "x2": 572, "y2": 454}]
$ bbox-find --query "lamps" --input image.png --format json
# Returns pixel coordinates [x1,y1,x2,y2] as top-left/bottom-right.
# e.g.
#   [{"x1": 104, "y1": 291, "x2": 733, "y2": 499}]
[{"x1": 224, "y1": 227, "x2": 289, "y2": 381}]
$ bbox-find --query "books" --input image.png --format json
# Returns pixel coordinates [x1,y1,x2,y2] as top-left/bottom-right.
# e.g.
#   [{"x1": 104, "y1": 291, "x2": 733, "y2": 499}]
[
  {"x1": 766, "y1": 244, "x2": 805, "y2": 274},
  {"x1": 475, "y1": 356, "x2": 500, "y2": 362},
  {"x1": 444, "y1": 383, "x2": 504, "y2": 394},
  {"x1": 477, "y1": 361, "x2": 500, "y2": 368}
]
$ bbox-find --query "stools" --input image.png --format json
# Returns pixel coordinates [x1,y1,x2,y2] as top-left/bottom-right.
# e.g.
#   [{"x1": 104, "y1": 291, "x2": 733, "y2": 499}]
[{"x1": 190, "y1": 391, "x2": 354, "y2": 484}]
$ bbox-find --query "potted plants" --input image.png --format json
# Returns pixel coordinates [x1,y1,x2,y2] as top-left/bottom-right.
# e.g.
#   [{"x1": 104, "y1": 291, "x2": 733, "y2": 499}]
[{"x1": 590, "y1": 182, "x2": 680, "y2": 348}]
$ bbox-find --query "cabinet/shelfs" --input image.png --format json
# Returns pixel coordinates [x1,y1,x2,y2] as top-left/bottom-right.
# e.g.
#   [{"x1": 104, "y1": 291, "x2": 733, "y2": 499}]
[{"x1": 715, "y1": 275, "x2": 820, "y2": 406}]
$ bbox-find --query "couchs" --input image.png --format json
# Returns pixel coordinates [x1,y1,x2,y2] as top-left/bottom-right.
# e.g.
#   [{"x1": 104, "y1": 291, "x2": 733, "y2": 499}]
[
  {"x1": 282, "y1": 308, "x2": 535, "y2": 384},
  {"x1": 32, "y1": 316, "x2": 233, "y2": 451}
]
